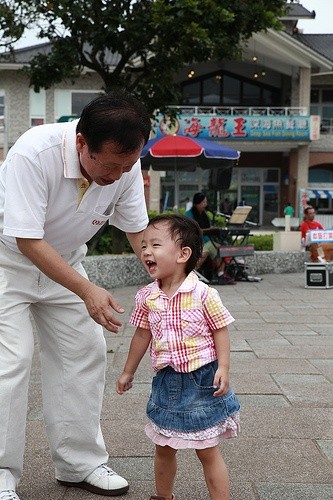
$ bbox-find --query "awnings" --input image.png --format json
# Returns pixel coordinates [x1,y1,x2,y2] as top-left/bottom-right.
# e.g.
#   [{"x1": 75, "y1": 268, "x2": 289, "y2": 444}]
[{"x1": 307, "y1": 188, "x2": 333, "y2": 199}]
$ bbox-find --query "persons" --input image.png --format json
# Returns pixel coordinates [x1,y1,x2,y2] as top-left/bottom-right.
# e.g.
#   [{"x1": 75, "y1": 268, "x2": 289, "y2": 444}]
[
  {"x1": 186, "y1": 197, "x2": 246, "y2": 215},
  {"x1": 116, "y1": 214, "x2": 240, "y2": 500},
  {"x1": 301, "y1": 208, "x2": 324, "y2": 251},
  {"x1": 0, "y1": 93, "x2": 152, "y2": 500},
  {"x1": 303, "y1": 201, "x2": 312, "y2": 213},
  {"x1": 184, "y1": 193, "x2": 235, "y2": 283},
  {"x1": 284, "y1": 203, "x2": 294, "y2": 234}
]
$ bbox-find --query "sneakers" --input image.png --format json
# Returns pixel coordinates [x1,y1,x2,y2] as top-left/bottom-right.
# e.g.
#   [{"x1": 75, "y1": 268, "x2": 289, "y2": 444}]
[
  {"x1": 0, "y1": 490, "x2": 20, "y2": 500},
  {"x1": 59, "y1": 464, "x2": 129, "y2": 496}
]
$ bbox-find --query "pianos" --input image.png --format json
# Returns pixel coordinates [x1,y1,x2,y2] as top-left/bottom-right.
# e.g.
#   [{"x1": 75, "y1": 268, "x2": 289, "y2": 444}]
[{"x1": 201, "y1": 227, "x2": 251, "y2": 236}]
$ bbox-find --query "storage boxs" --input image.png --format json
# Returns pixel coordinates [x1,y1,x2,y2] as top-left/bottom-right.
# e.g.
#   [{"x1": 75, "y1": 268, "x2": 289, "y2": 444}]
[{"x1": 304, "y1": 260, "x2": 333, "y2": 288}]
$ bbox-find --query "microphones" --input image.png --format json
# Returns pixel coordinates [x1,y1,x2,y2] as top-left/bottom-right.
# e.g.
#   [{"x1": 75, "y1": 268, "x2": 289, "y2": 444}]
[{"x1": 205, "y1": 207, "x2": 216, "y2": 215}]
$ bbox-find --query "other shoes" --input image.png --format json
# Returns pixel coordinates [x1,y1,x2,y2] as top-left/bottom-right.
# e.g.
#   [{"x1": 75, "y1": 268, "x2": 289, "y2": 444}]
[
  {"x1": 218, "y1": 273, "x2": 234, "y2": 283},
  {"x1": 150, "y1": 492, "x2": 175, "y2": 500}
]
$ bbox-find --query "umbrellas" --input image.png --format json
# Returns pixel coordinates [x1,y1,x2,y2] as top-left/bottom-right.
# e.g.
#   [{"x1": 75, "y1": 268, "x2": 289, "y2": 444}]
[{"x1": 140, "y1": 134, "x2": 240, "y2": 213}]
